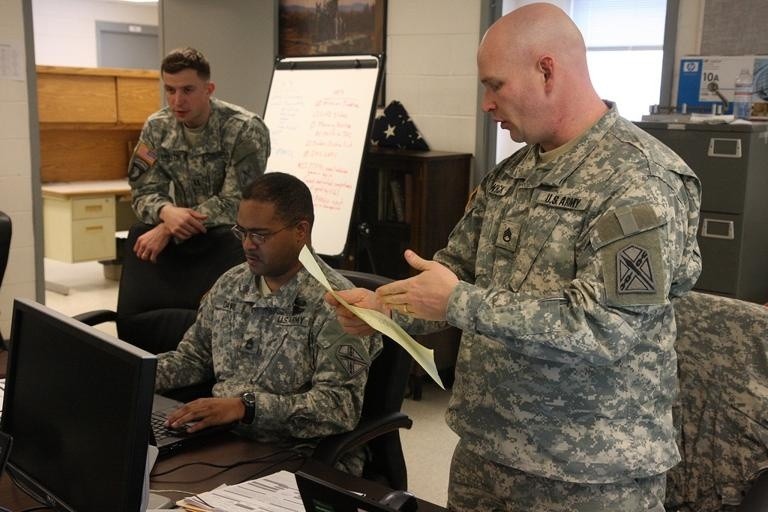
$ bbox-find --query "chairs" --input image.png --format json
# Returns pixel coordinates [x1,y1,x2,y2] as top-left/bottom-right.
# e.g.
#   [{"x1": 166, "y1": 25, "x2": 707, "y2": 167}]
[
  {"x1": 324, "y1": 266, "x2": 418, "y2": 491},
  {"x1": 70, "y1": 220, "x2": 245, "y2": 354}
]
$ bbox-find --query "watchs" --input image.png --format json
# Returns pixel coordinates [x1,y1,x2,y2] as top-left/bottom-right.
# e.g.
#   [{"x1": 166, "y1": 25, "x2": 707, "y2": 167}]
[{"x1": 240, "y1": 392, "x2": 256, "y2": 424}]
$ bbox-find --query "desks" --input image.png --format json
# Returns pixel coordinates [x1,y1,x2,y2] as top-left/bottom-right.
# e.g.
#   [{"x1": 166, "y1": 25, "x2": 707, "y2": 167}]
[{"x1": 3, "y1": 343, "x2": 450, "y2": 510}]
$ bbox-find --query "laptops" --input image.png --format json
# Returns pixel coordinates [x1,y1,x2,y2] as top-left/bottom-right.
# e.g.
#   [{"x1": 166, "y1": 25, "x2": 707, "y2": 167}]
[{"x1": 150, "y1": 393, "x2": 231, "y2": 462}]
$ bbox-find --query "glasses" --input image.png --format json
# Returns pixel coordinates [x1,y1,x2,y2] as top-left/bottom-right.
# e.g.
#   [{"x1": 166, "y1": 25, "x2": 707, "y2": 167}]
[{"x1": 231, "y1": 222, "x2": 301, "y2": 245}]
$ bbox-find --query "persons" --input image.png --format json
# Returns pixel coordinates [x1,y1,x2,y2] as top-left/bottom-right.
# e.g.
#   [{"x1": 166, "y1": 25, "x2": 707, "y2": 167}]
[
  {"x1": 154, "y1": 171, "x2": 385, "y2": 511},
  {"x1": 323, "y1": 2, "x2": 703, "y2": 511},
  {"x1": 126, "y1": 47, "x2": 272, "y2": 265}
]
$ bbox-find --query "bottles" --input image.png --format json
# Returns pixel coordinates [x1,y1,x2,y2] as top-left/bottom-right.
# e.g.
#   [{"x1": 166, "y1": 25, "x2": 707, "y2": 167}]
[{"x1": 732, "y1": 68, "x2": 751, "y2": 121}]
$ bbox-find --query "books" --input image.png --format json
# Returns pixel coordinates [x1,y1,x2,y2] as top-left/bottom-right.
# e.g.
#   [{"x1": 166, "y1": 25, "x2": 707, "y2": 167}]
[
  {"x1": 175, "y1": 468, "x2": 305, "y2": 511},
  {"x1": 642, "y1": 111, "x2": 735, "y2": 123},
  {"x1": 377, "y1": 162, "x2": 425, "y2": 226}
]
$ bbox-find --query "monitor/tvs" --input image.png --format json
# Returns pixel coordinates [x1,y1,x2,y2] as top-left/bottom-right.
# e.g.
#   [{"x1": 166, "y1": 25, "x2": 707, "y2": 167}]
[{"x1": 0, "y1": 297, "x2": 159, "y2": 512}]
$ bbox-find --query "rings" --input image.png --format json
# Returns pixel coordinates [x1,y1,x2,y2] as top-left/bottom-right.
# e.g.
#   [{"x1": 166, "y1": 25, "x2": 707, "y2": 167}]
[{"x1": 403, "y1": 302, "x2": 411, "y2": 314}]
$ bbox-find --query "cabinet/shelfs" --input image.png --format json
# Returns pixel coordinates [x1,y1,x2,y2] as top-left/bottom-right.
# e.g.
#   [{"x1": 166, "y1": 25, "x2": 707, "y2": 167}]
[
  {"x1": 643, "y1": 124, "x2": 767, "y2": 302},
  {"x1": 44, "y1": 192, "x2": 119, "y2": 265},
  {"x1": 361, "y1": 150, "x2": 472, "y2": 399}
]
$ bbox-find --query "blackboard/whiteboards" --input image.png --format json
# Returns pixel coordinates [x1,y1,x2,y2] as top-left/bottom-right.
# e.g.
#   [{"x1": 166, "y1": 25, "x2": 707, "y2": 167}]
[{"x1": 262, "y1": 53, "x2": 383, "y2": 255}]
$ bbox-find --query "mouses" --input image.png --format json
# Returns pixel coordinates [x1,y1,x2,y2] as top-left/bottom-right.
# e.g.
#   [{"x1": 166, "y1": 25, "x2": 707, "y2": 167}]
[{"x1": 378, "y1": 490, "x2": 414, "y2": 511}]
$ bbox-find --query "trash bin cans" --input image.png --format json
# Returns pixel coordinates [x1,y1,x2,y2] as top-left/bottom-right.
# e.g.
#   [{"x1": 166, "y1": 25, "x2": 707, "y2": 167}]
[{"x1": 103, "y1": 239, "x2": 125, "y2": 280}]
